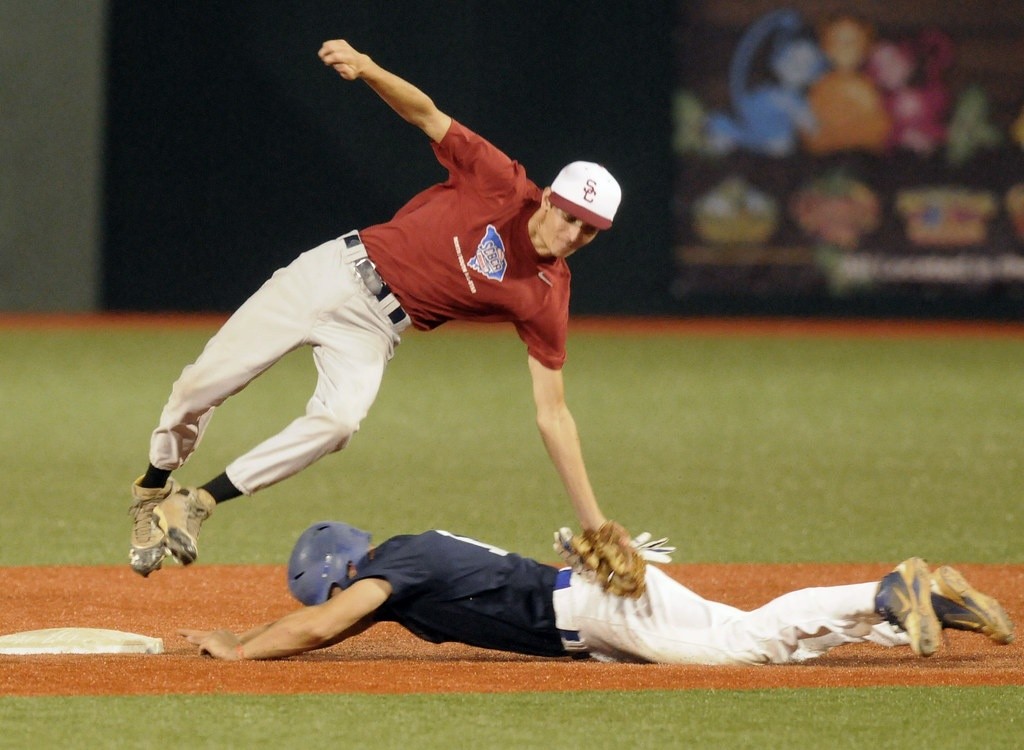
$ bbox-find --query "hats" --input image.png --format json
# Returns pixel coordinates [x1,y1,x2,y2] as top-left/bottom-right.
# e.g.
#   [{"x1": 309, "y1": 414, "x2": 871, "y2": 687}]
[{"x1": 549, "y1": 160, "x2": 621, "y2": 230}]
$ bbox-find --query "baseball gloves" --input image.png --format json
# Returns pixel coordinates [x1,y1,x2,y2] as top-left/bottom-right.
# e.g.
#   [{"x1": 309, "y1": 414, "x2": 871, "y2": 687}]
[{"x1": 570, "y1": 519, "x2": 647, "y2": 601}]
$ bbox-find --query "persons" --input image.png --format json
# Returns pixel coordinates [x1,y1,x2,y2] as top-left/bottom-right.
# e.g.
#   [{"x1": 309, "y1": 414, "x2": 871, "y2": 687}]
[
  {"x1": 178, "y1": 522, "x2": 1012, "y2": 666},
  {"x1": 127, "y1": 39, "x2": 646, "y2": 600}
]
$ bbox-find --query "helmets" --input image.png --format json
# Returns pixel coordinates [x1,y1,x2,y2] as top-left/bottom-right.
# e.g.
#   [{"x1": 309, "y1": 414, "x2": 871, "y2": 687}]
[{"x1": 288, "y1": 522, "x2": 371, "y2": 606}]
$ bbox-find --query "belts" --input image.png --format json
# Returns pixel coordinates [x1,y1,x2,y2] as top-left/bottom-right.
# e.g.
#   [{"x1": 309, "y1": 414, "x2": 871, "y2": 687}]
[{"x1": 344, "y1": 235, "x2": 407, "y2": 325}]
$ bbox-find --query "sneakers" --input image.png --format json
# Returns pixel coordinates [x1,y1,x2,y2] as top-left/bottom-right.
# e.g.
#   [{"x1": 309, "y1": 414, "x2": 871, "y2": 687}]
[
  {"x1": 130, "y1": 474, "x2": 180, "y2": 577},
  {"x1": 152, "y1": 486, "x2": 216, "y2": 567},
  {"x1": 875, "y1": 557, "x2": 943, "y2": 657},
  {"x1": 930, "y1": 565, "x2": 1014, "y2": 644}
]
